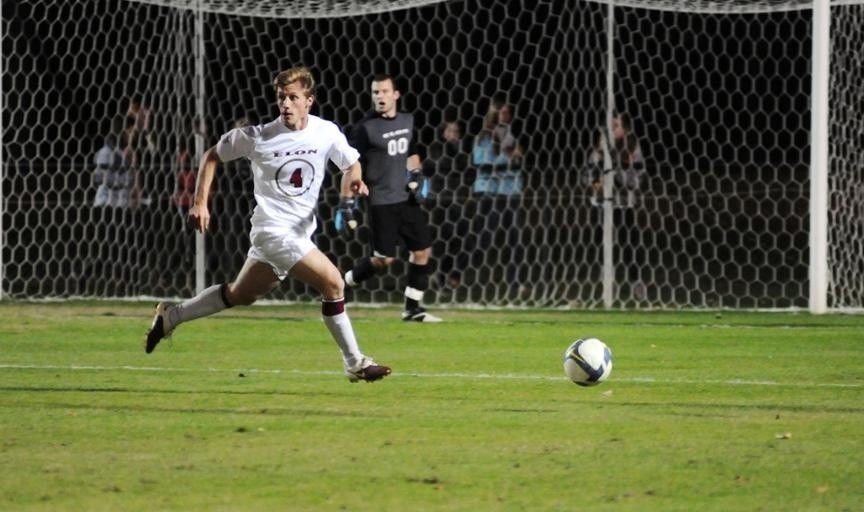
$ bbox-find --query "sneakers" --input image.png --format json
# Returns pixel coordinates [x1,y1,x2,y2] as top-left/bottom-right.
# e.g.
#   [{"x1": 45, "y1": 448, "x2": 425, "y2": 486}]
[
  {"x1": 144, "y1": 301, "x2": 179, "y2": 354},
  {"x1": 344, "y1": 354, "x2": 392, "y2": 383},
  {"x1": 401, "y1": 306, "x2": 444, "y2": 323}
]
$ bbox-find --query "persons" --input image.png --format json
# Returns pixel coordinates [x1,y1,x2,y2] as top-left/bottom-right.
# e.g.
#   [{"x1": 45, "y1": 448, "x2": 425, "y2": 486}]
[
  {"x1": 468, "y1": 98, "x2": 526, "y2": 198},
  {"x1": 342, "y1": 74, "x2": 446, "y2": 323},
  {"x1": 427, "y1": 122, "x2": 471, "y2": 193},
  {"x1": 581, "y1": 109, "x2": 648, "y2": 210},
  {"x1": 87, "y1": 98, "x2": 255, "y2": 233},
  {"x1": 144, "y1": 65, "x2": 394, "y2": 382}
]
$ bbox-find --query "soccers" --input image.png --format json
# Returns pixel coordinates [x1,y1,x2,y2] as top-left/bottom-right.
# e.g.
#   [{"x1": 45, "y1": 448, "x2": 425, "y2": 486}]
[{"x1": 564, "y1": 337, "x2": 612, "y2": 386}]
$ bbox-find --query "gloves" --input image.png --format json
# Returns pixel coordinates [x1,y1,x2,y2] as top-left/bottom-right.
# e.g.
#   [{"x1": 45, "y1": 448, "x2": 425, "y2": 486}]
[
  {"x1": 405, "y1": 169, "x2": 429, "y2": 204},
  {"x1": 334, "y1": 198, "x2": 358, "y2": 242}
]
